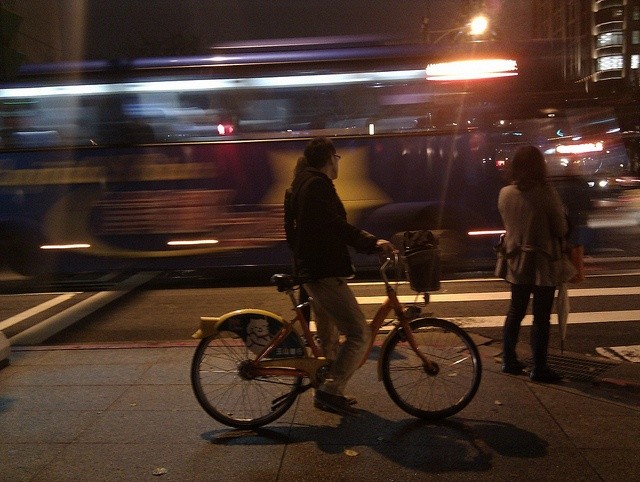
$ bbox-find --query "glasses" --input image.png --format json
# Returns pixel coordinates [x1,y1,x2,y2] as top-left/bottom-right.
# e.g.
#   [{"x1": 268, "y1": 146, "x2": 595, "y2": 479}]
[{"x1": 330, "y1": 153, "x2": 346, "y2": 161}]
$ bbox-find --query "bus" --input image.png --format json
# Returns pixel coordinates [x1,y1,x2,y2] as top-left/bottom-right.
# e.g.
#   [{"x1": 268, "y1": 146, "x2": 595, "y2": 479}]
[{"x1": 1, "y1": 33, "x2": 622, "y2": 285}]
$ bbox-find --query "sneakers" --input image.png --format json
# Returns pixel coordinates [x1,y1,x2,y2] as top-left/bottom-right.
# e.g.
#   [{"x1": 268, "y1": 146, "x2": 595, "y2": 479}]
[
  {"x1": 502, "y1": 359, "x2": 525, "y2": 374},
  {"x1": 529, "y1": 367, "x2": 563, "y2": 381}
]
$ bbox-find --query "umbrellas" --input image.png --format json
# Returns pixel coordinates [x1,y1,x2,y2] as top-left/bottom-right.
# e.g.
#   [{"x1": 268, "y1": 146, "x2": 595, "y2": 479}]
[{"x1": 555, "y1": 237, "x2": 569, "y2": 358}]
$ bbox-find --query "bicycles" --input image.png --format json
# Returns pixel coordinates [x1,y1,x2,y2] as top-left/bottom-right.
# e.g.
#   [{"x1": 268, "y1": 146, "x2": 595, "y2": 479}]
[{"x1": 190, "y1": 248, "x2": 483, "y2": 431}]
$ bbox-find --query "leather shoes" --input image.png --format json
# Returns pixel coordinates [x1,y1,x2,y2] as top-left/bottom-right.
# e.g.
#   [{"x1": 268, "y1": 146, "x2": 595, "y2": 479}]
[{"x1": 311, "y1": 391, "x2": 363, "y2": 418}]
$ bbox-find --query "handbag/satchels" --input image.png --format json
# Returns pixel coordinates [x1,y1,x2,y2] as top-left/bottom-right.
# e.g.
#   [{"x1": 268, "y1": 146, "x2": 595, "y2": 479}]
[{"x1": 564, "y1": 243, "x2": 584, "y2": 281}]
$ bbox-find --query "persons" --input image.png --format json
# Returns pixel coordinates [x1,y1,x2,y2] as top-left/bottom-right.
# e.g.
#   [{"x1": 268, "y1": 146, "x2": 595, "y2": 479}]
[
  {"x1": 495, "y1": 146, "x2": 581, "y2": 382},
  {"x1": 290, "y1": 138, "x2": 397, "y2": 418},
  {"x1": 281, "y1": 156, "x2": 329, "y2": 344}
]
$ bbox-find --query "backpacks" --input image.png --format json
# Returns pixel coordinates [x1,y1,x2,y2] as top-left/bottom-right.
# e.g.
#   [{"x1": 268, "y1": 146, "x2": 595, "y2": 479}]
[{"x1": 404, "y1": 230, "x2": 439, "y2": 288}]
